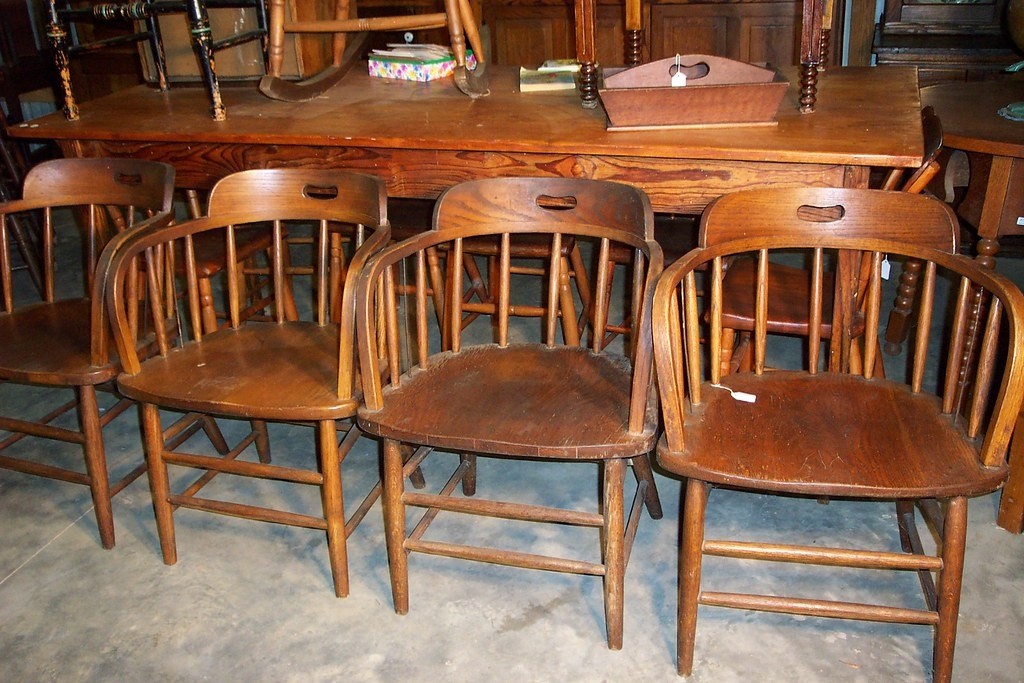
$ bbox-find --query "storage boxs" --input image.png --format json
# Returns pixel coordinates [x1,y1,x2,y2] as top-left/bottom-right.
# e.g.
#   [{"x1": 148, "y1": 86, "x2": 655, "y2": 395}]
[{"x1": 369, "y1": 50, "x2": 478, "y2": 82}]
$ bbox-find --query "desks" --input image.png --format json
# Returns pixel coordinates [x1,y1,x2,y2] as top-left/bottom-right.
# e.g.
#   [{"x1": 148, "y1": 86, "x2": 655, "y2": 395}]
[
  {"x1": 8, "y1": 65, "x2": 923, "y2": 374},
  {"x1": 871, "y1": 0, "x2": 1024, "y2": 89},
  {"x1": 919, "y1": 81, "x2": 1024, "y2": 416}
]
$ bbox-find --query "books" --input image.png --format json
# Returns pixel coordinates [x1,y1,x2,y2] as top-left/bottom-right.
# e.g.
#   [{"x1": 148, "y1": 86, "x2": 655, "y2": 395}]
[{"x1": 520, "y1": 60, "x2": 582, "y2": 90}]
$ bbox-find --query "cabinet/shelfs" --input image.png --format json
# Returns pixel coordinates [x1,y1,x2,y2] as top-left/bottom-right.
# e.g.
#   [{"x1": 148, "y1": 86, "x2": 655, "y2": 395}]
[{"x1": 63, "y1": 0, "x2": 876, "y2": 103}]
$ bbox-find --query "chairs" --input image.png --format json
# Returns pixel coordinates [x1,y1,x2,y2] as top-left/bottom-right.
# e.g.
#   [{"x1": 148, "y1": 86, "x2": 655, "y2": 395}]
[
  {"x1": 259, "y1": 0, "x2": 493, "y2": 103},
  {"x1": 574, "y1": 1, "x2": 836, "y2": 114},
  {"x1": 651, "y1": 187, "x2": 1023, "y2": 683},
  {"x1": 357, "y1": 176, "x2": 662, "y2": 650},
  {"x1": 705, "y1": 107, "x2": 945, "y2": 379},
  {"x1": 0, "y1": 158, "x2": 230, "y2": 549},
  {"x1": 108, "y1": 169, "x2": 427, "y2": 598},
  {"x1": 47, "y1": 0, "x2": 269, "y2": 122},
  {"x1": 0, "y1": 16, "x2": 736, "y2": 348}
]
